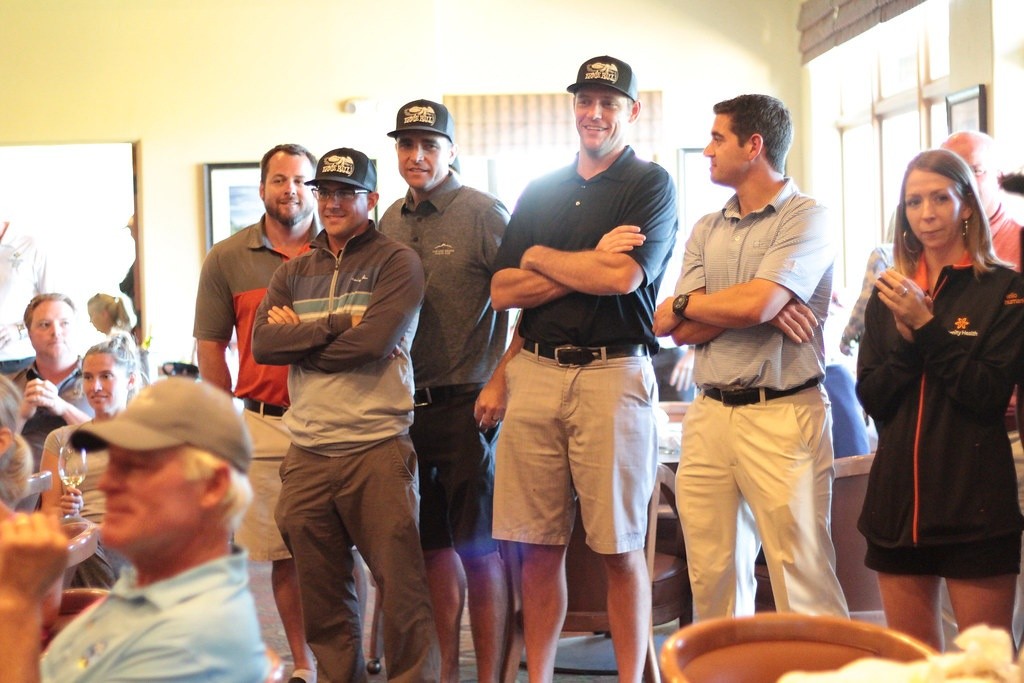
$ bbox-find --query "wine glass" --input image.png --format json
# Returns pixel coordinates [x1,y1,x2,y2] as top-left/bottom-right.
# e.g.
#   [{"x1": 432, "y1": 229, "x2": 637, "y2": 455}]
[{"x1": 58, "y1": 447, "x2": 87, "y2": 518}]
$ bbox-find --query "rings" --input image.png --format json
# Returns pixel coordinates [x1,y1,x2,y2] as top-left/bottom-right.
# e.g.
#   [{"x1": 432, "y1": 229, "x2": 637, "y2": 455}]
[{"x1": 900, "y1": 289, "x2": 907, "y2": 296}]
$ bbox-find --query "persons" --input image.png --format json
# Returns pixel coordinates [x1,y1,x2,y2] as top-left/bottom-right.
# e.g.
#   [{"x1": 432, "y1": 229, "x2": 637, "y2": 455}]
[
  {"x1": 0, "y1": 102, "x2": 525, "y2": 683},
  {"x1": 652, "y1": 133, "x2": 1024, "y2": 664},
  {"x1": 486, "y1": 57, "x2": 679, "y2": 683},
  {"x1": 651, "y1": 95, "x2": 853, "y2": 622}
]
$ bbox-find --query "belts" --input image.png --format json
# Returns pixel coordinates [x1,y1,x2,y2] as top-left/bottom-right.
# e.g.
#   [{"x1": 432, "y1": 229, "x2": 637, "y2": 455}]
[
  {"x1": 415, "y1": 380, "x2": 485, "y2": 406},
  {"x1": 523, "y1": 340, "x2": 647, "y2": 366},
  {"x1": 704, "y1": 378, "x2": 818, "y2": 407},
  {"x1": 244, "y1": 396, "x2": 289, "y2": 417}
]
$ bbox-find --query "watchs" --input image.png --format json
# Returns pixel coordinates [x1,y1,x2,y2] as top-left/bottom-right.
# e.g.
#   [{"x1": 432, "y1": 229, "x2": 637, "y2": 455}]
[{"x1": 672, "y1": 293, "x2": 691, "y2": 319}]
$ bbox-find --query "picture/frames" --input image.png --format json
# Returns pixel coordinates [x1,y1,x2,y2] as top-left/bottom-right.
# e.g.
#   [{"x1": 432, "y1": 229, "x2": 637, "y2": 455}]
[{"x1": 203, "y1": 160, "x2": 380, "y2": 253}]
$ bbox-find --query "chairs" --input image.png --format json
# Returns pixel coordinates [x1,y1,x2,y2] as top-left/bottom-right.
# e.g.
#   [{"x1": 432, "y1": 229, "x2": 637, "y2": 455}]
[
  {"x1": 497, "y1": 454, "x2": 694, "y2": 683},
  {"x1": 755, "y1": 451, "x2": 882, "y2": 611},
  {"x1": 659, "y1": 610, "x2": 943, "y2": 683}
]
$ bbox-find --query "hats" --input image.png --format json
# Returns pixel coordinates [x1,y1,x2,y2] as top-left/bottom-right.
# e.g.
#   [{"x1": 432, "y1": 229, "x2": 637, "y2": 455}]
[
  {"x1": 71, "y1": 378, "x2": 253, "y2": 474},
  {"x1": 388, "y1": 99, "x2": 457, "y2": 143},
  {"x1": 449, "y1": 156, "x2": 459, "y2": 174},
  {"x1": 306, "y1": 147, "x2": 377, "y2": 191},
  {"x1": 568, "y1": 57, "x2": 638, "y2": 103}
]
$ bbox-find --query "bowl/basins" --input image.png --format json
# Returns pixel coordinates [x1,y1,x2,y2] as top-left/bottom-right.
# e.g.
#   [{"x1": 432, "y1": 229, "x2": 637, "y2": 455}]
[{"x1": 660, "y1": 612, "x2": 940, "y2": 683}]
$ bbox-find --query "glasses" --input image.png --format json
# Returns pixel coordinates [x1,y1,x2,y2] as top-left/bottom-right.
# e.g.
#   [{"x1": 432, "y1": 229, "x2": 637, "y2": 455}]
[
  {"x1": 971, "y1": 165, "x2": 997, "y2": 178},
  {"x1": 310, "y1": 188, "x2": 370, "y2": 200}
]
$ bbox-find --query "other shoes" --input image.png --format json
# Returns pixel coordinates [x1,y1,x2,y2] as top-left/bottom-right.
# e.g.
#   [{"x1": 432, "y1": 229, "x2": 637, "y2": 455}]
[{"x1": 289, "y1": 669, "x2": 316, "y2": 683}]
